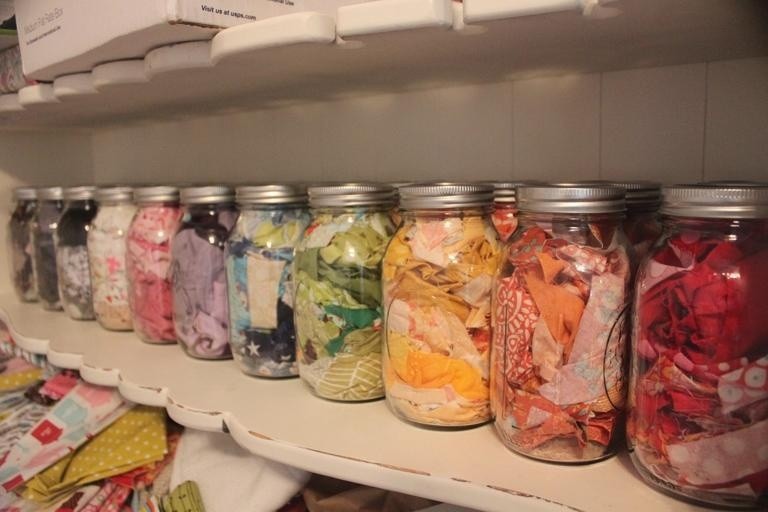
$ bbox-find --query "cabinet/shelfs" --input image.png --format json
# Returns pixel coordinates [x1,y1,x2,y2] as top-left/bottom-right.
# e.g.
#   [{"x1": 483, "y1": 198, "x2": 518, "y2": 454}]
[{"x1": 0, "y1": 0, "x2": 768, "y2": 511}]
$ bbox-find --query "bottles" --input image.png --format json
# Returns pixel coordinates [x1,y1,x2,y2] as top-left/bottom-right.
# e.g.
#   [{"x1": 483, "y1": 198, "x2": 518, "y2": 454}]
[
  {"x1": 627, "y1": 177, "x2": 768, "y2": 509},
  {"x1": 291, "y1": 180, "x2": 398, "y2": 406},
  {"x1": 9, "y1": 175, "x2": 307, "y2": 382},
  {"x1": 490, "y1": 179, "x2": 625, "y2": 467},
  {"x1": 376, "y1": 181, "x2": 505, "y2": 437}
]
